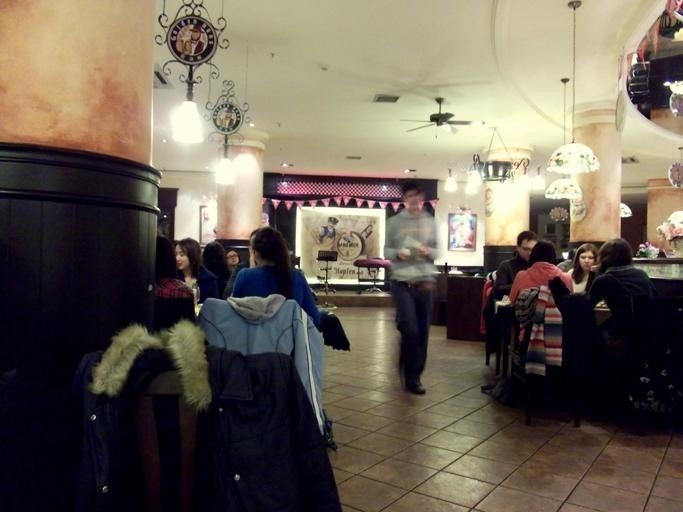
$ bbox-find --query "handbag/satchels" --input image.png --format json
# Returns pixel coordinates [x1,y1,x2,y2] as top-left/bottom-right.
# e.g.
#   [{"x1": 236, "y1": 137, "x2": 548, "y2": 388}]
[{"x1": 480, "y1": 376, "x2": 515, "y2": 405}]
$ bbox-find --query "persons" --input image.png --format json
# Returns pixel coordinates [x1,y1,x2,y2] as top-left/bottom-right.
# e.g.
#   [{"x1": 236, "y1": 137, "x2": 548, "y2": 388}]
[
  {"x1": 154, "y1": 229, "x2": 327, "y2": 330},
  {"x1": 492, "y1": 229, "x2": 663, "y2": 404},
  {"x1": 313, "y1": 216, "x2": 340, "y2": 247},
  {"x1": 384, "y1": 185, "x2": 440, "y2": 397}
]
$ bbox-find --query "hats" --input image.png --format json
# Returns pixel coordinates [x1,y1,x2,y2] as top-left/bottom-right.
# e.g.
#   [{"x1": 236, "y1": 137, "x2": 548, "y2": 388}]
[{"x1": 513, "y1": 287, "x2": 540, "y2": 322}]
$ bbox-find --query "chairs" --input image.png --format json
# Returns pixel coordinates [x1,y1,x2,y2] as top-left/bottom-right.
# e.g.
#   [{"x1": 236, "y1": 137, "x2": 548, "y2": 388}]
[
  {"x1": 484, "y1": 259, "x2": 683, "y2": 427},
  {"x1": 315, "y1": 250, "x2": 337, "y2": 294}
]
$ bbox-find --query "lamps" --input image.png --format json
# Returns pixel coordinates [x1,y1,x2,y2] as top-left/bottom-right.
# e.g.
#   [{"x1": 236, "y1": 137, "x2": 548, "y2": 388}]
[{"x1": 543, "y1": 1, "x2": 600, "y2": 202}]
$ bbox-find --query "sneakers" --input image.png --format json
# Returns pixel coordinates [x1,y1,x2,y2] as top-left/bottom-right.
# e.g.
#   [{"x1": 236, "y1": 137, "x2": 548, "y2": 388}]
[{"x1": 405, "y1": 375, "x2": 425, "y2": 393}]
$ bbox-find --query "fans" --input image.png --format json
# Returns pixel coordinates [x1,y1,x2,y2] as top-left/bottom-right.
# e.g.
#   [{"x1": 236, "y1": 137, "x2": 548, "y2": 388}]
[{"x1": 401, "y1": 97, "x2": 484, "y2": 135}]
